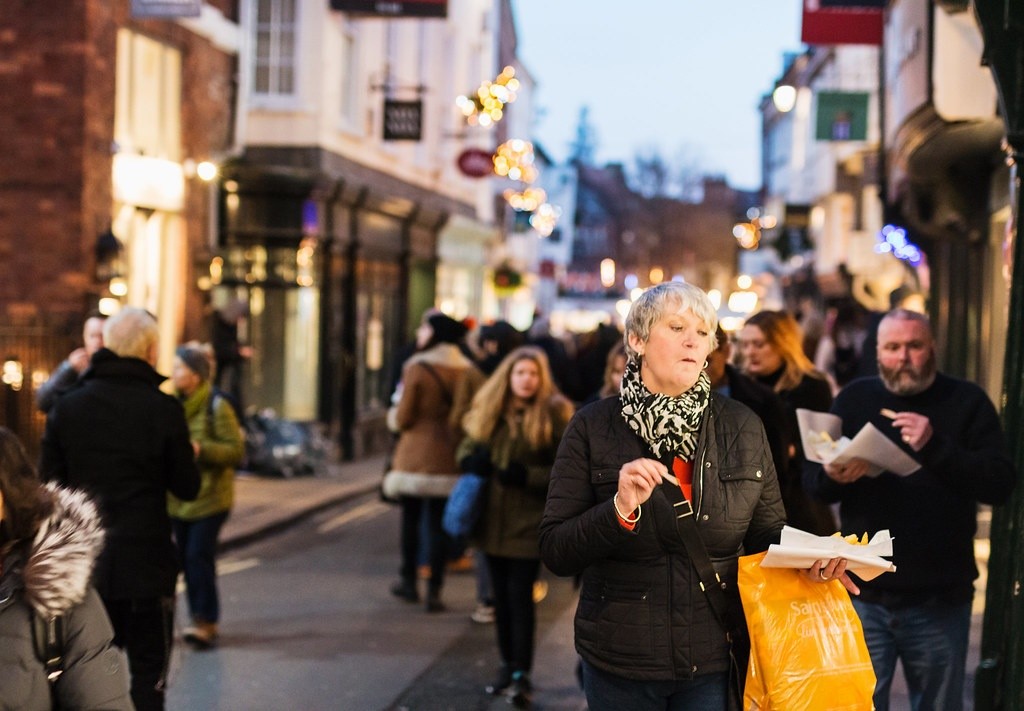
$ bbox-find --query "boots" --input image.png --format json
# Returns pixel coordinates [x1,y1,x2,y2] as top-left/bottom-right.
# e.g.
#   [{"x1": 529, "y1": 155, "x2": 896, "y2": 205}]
[
  {"x1": 423, "y1": 579, "x2": 444, "y2": 610},
  {"x1": 390, "y1": 572, "x2": 419, "y2": 600}
]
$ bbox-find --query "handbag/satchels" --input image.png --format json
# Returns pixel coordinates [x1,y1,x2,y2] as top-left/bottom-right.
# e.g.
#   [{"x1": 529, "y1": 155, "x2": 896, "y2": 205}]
[
  {"x1": 442, "y1": 472, "x2": 488, "y2": 540},
  {"x1": 728, "y1": 636, "x2": 748, "y2": 711},
  {"x1": 736, "y1": 532, "x2": 878, "y2": 711}
]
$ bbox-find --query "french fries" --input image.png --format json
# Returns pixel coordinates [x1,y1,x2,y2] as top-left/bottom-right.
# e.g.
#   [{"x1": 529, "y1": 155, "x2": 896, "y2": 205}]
[
  {"x1": 832, "y1": 531, "x2": 868, "y2": 544},
  {"x1": 881, "y1": 409, "x2": 897, "y2": 419},
  {"x1": 820, "y1": 430, "x2": 833, "y2": 444}
]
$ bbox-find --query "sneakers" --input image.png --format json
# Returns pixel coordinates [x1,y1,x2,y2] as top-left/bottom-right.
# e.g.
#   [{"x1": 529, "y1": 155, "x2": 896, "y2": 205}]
[{"x1": 181, "y1": 620, "x2": 217, "y2": 646}]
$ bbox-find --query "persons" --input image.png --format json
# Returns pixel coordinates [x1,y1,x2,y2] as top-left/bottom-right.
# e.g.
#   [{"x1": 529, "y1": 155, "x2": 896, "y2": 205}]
[
  {"x1": 0, "y1": 428, "x2": 135, "y2": 711},
  {"x1": 211, "y1": 299, "x2": 255, "y2": 424},
  {"x1": 158, "y1": 340, "x2": 246, "y2": 645},
  {"x1": 35, "y1": 312, "x2": 107, "y2": 413},
  {"x1": 454, "y1": 347, "x2": 574, "y2": 705},
  {"x1": 535, "y1": 281, "x2": 860, "y2": 710},
  {"x1": 41, "y1": 307, "x2": 201, "y2": 711},
  {"x1": 377, "y1": 306, "x2": 624, "y2": 620},
  {"x1": 789, "y1": 308, "x2": 1009, "y2": 711},
  {"x1": 703, "y1": 295, "x2": 860, "y2": 537}
]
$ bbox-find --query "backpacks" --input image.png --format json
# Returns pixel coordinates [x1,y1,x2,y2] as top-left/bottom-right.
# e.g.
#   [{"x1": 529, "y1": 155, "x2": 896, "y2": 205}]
[{"x1": 418, "y1": 361, "x2": 465, "y2": 447}]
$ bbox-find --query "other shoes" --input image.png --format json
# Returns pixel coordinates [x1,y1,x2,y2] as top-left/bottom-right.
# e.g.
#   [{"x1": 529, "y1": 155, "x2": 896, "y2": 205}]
[
  {"x1": 533, "y1": 577, "x2": 548, "y2": 601},
  {"x1": 510, "y1": 674, "x2": 532, "y2": 699},
  {"x1": 449, "y1": 552, "x2": 476, "y2": 571},
  {"x1": 470, "y1": 603, "x2": 495, "y2": 624},
  {"x1": 486, "y1": 665, "x2": 513, "y2": 698}
]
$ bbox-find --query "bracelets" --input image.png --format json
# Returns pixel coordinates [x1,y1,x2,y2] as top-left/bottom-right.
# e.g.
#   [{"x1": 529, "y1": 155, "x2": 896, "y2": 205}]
[{"x1": 614, "y1": 491, "x2": 642, "y2": 522}]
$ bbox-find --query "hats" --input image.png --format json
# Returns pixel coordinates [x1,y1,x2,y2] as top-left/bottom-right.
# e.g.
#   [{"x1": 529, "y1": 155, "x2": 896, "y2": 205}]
[{"x1": 175, "y1": 344, "x2": 214, "y2": 380}]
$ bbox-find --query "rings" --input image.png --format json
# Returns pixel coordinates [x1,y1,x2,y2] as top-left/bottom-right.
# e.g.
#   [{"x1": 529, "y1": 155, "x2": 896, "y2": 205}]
[
  {"x1": 820, "y1": 570, "x2": 827, "y2": 580},
  {"x1": 905, "y1": 434, "x2": 910, "y2": 444}
]
